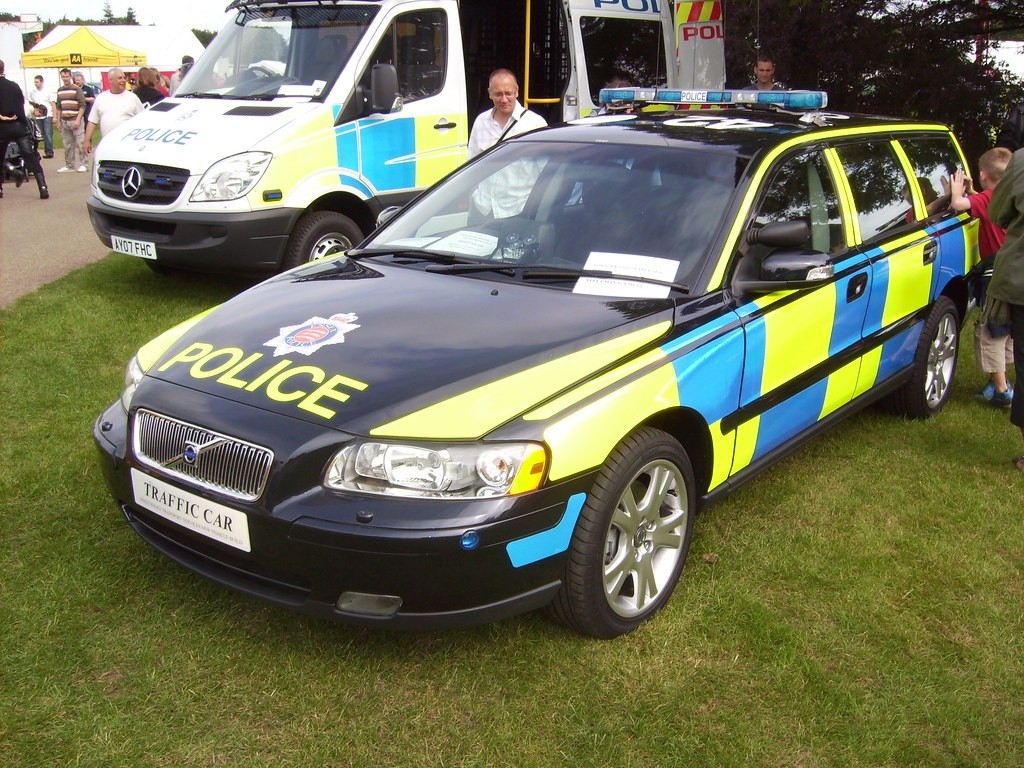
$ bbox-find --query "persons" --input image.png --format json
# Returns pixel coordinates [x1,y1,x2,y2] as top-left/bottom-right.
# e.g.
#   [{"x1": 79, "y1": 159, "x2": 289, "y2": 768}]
[
  {"x1": 3, "y1": 158, "x2": 26, "y2": 187},
  {"x1": 741, "y1": 56, "x2": 785, "y2": 90},
  {"x1": 946, "y1": 146, "x2": 1024, "y2": 472},
  {"x1": 465, "y1": 67, "x2": 553, "y2": 227},
  {"x1": 568, "y1": 77, "x2": 659, "y2": 204},
  {"x1": 902, "y1": 174, "x2": 952, "y2": 225},
  {"x1": 0, "y1": 60, "x2": 50, "y2": 200},
  {"x1": 22, "y1": 55, "x2": 196, "y2": 173}
]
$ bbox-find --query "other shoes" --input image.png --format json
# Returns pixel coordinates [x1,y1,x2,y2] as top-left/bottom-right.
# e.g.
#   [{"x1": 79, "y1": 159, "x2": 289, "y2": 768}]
[
  {"x1": 40, "y1": 187, "x2": 49, "y2": 199},
  {"x1": 43, "y1": 155, "x2": 54, "y2": 158},
  {"x1": 14, "y1": 168, "x2": 25, "y2": 188},
  {"x1": 974, "y1": 378, "x2": 1013, "y2": 409},
  {"x1": 1013, "y1": 456, "x2": 1024, "y2": 472}
]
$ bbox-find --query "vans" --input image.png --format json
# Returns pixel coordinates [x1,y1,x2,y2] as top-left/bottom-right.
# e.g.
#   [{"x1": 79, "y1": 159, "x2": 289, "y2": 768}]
[{"x1": 86, "y1": 0, "x2": 727, "y2": 282}]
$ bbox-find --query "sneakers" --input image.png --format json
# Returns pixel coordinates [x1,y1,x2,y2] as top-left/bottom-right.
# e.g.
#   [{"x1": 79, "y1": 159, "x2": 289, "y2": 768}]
[
  {"x1": 77, "y1": 165, "x2": 88, "y2": 173},
  {"x1": 57, "y1": 167, "x2": 77, "y2": 173}
]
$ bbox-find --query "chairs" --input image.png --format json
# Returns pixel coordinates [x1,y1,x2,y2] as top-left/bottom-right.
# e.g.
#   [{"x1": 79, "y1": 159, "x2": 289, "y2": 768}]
[{"x1": 302, "y1": 35, "x2": 346, "y2": 90}]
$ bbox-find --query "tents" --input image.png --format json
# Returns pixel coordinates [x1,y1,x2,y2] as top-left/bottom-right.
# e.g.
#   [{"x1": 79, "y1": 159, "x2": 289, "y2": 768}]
[
  {"x1": 24, "y1": 24, "x2": 207, "y2": 102},
  {"x1": 20, "y1": 26, "x2": 148, "y2": 98}
]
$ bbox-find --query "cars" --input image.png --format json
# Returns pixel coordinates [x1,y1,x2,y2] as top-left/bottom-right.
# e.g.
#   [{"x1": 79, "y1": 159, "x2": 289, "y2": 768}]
[{"x1": 92, "y1": 89, "x2": 987, "y2": 639}]
